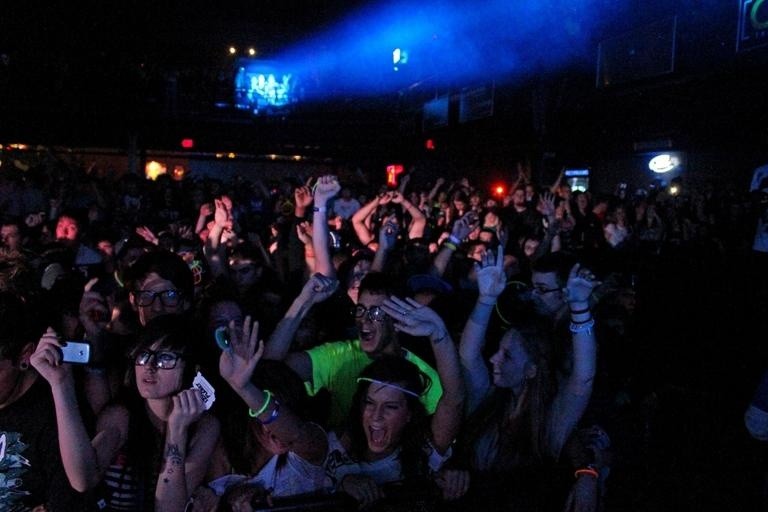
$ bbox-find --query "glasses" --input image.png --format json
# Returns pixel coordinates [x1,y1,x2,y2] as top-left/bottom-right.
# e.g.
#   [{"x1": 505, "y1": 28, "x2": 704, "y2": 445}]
[
  {"x1": 134, "y1": 289, "x2": 183, "y2": 307},
  {"x1": 134, "y1": 348, "x2": 182, "y2": 370},
  {"x1": 351, "y1": 303, "x2": 386, "y2": 322},
  {"x1": 534, "y1": 285, "x2": 562, "y2": 295}
]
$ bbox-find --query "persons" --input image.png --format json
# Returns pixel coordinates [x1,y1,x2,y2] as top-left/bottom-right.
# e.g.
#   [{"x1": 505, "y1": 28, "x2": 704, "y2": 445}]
[{"x1": 0, "y1": 159, "x2": 768, "y2": 512}]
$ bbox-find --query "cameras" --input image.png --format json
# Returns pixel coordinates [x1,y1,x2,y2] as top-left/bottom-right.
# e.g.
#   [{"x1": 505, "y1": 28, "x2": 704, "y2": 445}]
[{"x1": 59, "y1": 340, "x2": 92, "y2": 365}]
